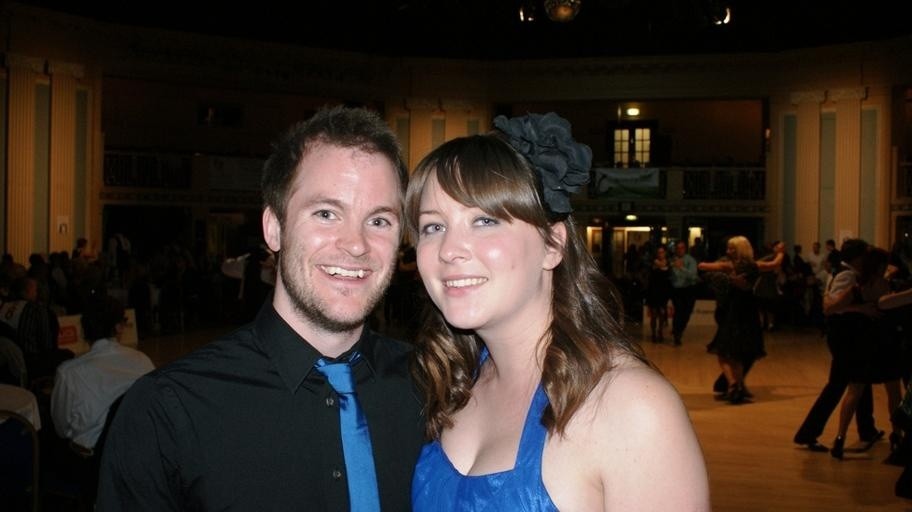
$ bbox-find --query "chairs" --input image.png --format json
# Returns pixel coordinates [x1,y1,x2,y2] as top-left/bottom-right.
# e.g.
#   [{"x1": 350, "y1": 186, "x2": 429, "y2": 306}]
[{"x1": 3, "y1": 274, "x2": 189, "y2": 509}]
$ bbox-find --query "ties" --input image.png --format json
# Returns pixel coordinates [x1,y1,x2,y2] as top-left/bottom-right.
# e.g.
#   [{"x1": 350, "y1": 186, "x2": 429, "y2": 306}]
[{"x1": 314, "y1": 348, "x2": 384, "y2": 512}]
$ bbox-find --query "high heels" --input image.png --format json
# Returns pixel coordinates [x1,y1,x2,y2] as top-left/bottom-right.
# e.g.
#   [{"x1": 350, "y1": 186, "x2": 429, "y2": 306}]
[
  {"x1": 889, "y1": 431, "x2": 904, "y2": 450},
  {"x1": 830, "y1": 434, "x2": 844, "y2": 460}
]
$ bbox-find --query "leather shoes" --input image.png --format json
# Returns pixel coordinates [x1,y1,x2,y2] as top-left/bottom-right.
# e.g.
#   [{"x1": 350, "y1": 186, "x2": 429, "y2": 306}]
[
  {"x1": 649, "y1": 331, "x2": 682, "y2": 347},
  {"x1": 858, "y1": 428, "x2": 886, "y2": 441},
  {"x1": 712, "y1": 372, "x2": 755, "y2": 406},
  {"x1": 793, "y1": 433, "x2": 828, "y2": 453}
]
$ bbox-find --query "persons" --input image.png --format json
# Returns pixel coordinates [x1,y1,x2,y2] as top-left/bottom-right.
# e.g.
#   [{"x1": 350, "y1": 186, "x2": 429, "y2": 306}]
[
  {"x1": 237, "y1": 234, "x2": 276, "y2": 315},
  {"x1": 794, "y1": 235, "x2": 912, "y2": 500},
  {"x1": 640, "y1": 240, "x2": 701, "y2": 345},
  {"x1": 390, "y1": 248, "x2": 418, "y2": 284},
  {"x1": 405, "y1": 111, "x2": 711, "y2": 512},
  {"x1": 759, "y1": 239, "x2": 841, "y2": 333},
  {"x1": 2, "y1": 238, "x2": 96, "y2": 386},
  {"x1": 697, "y1": 236, "x2": 785, "y2": 403},
  {"x1": 109, "y1": 234, "x2": 195, "y2": 289},
  {"x1": 49, "y1": 294, "x2": 158, "y2": 450},
  {"x1": 96, "y1": 103, "x2": 428, "y2": 512}
]
